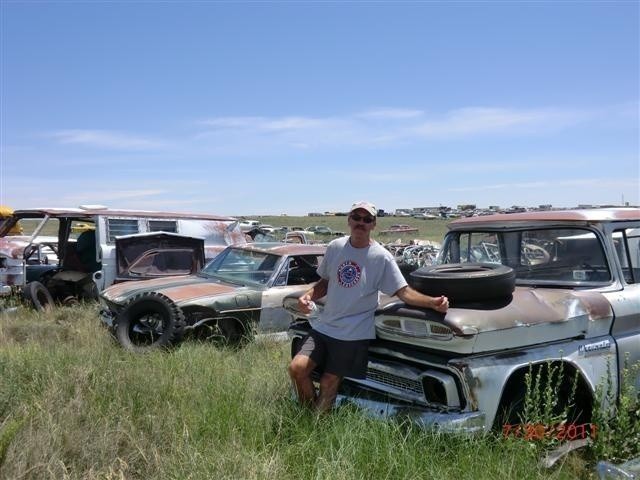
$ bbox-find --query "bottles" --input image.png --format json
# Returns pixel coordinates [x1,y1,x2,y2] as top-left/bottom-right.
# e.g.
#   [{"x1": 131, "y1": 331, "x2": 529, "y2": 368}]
[
  {"x1": 304, "y1": 300, "x2": 323, "y2": 329},
  {"x1": 595, "y1": 460, "x2": 634, "y2": 480}
]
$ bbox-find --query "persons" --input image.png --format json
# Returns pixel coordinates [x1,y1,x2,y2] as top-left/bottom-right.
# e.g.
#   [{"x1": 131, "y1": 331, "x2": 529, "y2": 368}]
[{"x1": 286, "y1": 197, "x2": 450, "y2": 415}]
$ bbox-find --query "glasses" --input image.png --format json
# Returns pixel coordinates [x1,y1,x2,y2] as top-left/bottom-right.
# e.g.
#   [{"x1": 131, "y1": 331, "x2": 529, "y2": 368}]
[{"x1": 350, "y1": 215, "x2": 374, "y2": 224}]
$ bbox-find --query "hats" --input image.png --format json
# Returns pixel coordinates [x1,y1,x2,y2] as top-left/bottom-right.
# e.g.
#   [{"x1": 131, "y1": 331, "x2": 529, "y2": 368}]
[{"x1": 350, "y1": 200, "x2": 378, "y2": 218}]
